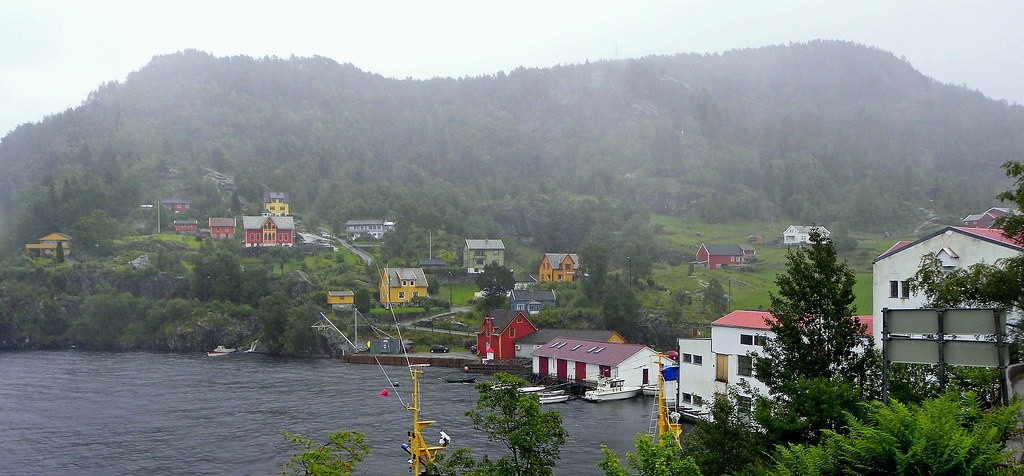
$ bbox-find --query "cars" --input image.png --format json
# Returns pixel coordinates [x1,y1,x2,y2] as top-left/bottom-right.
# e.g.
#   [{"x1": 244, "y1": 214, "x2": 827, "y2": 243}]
[
  {"x1": 429, "y1": 344, "x2": 449, "y2": 353},
  {"x1": 664, "y1": 351, "x2": 679, "y2": 360}
]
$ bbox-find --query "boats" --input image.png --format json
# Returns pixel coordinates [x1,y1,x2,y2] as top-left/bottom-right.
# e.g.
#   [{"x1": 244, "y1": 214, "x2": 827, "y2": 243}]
[
  {"x1": 207, "y1": 352, "x2": 230, "y2": 356},
  {"x1": 445, "y1": 376, "x2": 476, "y2": 383},
  {"x1": 584, "y1": 379, "x2": 642, "y2": 403},
  {"x1": 491, "y1": 382, "x2": 569, "y2": 404},
  {"x1": 214, "y1": 345, "x2": 236, "y2": 353},
  {"x1": 643, "y1": 382, "x2": 678, "y2": 396}
]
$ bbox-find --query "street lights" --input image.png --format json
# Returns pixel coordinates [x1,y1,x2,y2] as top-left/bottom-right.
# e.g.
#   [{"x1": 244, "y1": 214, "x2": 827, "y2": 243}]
[
  {"x1": 426, "y1": 228, "x2": 432, "y2": 261},
  {"x1": 448, "y1": 271, "x2": 452, "y2": 306}
]
